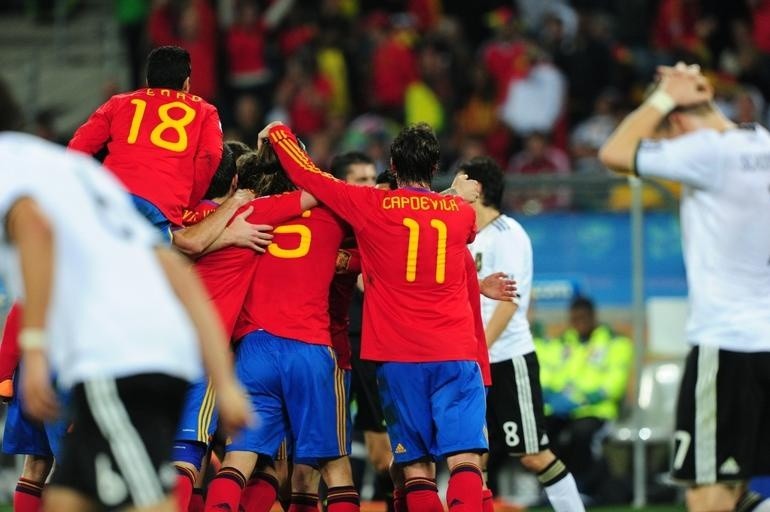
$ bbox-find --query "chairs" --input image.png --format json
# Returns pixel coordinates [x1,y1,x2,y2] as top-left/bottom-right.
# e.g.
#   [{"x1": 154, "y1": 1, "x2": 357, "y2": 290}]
[{"x1": 607, "y1": 359, "x2": 687, "y2": 503}]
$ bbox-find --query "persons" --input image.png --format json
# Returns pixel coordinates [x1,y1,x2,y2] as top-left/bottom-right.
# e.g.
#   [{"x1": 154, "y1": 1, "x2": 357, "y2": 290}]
[{"x1": 0, "y1": 0, "x2": 770, "y2": 512}]
